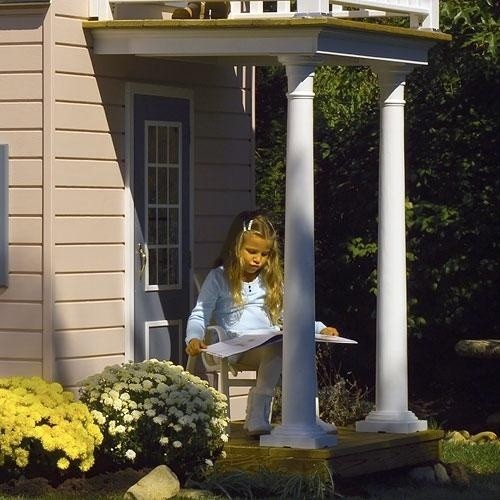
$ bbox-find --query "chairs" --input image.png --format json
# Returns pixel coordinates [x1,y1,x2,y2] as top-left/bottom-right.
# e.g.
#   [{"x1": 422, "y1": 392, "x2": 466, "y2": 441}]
[{"x1": 185, "y1": 268, "x2": 323, "y2": 435}]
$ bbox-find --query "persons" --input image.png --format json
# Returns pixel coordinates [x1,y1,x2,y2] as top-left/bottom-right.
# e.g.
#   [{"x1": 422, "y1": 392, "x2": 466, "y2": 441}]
[{"x1": 184, "y1": 208, "x2": 339, "y2": 434}]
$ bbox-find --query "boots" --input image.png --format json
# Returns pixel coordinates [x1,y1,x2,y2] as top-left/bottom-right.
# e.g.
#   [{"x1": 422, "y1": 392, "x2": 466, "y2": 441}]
[
  {"x1": 315, "y1": 396, "x2": 338, "y2": 435},
  {"x1": 243, "y1": 390, "x2": 271, "y2": 436}
]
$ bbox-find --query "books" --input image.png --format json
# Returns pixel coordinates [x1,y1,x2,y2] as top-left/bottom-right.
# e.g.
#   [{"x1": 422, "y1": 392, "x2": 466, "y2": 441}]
[{"x1": 200, "y1": 330, "x2": 357, "y2": 360}]
